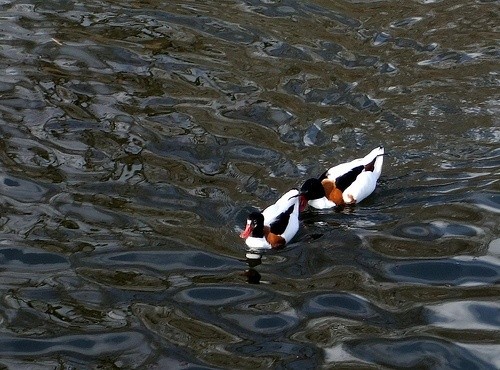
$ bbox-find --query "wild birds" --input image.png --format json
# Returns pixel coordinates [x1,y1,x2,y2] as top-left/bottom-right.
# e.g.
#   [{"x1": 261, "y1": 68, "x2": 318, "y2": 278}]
[
  {"x1": 288, "y1": 145, "x2": 384, "y2": 211},
  {"x1": 239, "y1": 188, "x2": 299, "y2": 248}
]
